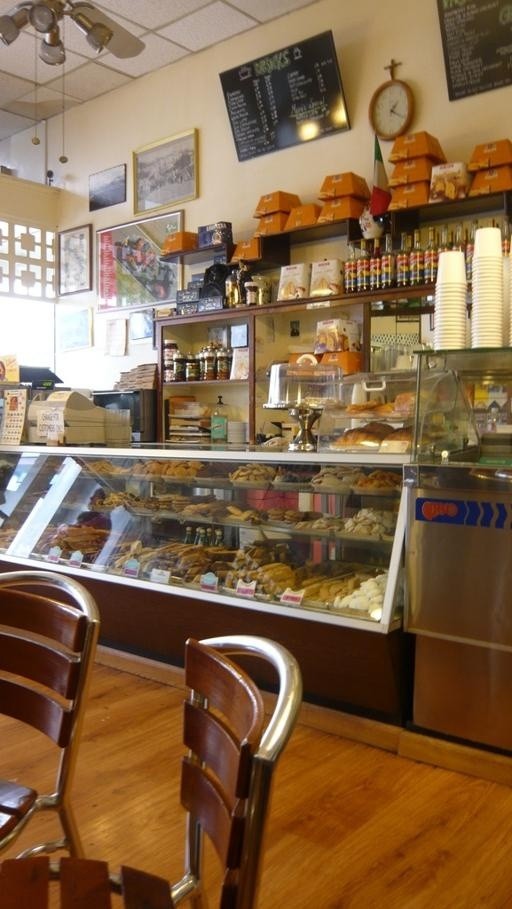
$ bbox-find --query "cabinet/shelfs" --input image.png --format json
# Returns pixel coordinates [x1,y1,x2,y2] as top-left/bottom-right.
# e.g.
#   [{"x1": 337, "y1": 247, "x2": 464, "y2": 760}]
[
  {"x1": 0, "y1": 444, "x2": 410, "y2": 623},
  {"x1": 156, "y1": 188, "x2": 512, "y2": 443}
]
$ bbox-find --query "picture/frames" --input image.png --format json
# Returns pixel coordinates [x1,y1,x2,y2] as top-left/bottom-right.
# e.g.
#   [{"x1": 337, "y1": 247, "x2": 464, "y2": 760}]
[
  {"x1": 134, "y1": 128, "x2": 200, "y2": 217},
  {"x1": 95, "y1": 209, "x2": 185, "y2": 321},
  {"x1": 55, "y1": 223, "x2": 92, "y2": 296}
]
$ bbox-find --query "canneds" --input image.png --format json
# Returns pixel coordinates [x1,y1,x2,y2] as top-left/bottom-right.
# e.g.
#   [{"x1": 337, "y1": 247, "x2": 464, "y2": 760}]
[{"x1": 163, "y1": 339, "x2": 178, "y2": 382}]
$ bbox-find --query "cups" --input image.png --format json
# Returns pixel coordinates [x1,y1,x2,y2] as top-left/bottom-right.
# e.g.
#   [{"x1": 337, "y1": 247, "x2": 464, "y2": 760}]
[
  {"x1": 433, "y1": 218, "x2": 512, "y2": 350},
  {"x1": 227, "y1": 419, "x2": 247, "y2": 442}
]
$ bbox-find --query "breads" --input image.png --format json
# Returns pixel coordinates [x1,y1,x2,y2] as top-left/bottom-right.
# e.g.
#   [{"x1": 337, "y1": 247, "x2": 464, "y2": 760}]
[{"x1": 330, "y1": 390, "x2": 445, "y2": 450}]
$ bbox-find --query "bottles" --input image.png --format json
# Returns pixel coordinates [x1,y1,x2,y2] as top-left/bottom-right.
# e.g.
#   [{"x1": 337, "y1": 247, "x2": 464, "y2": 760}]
[
  {"x1": 184, "y1": 524, "x2": 227, "y2": 547},
  {"x1": 211, "y1": 395, "x2": 232, "y2": 444},
  {"x1": 343, "y1": 219, "x2": 432, "y2": 290},
  {"x1": 163, "y1": 338, "x2": 233, "y2": 381}
]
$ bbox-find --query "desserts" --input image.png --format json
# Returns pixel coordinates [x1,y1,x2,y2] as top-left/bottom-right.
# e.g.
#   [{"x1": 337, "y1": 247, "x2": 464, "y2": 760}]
[{"x1": 0, "y1": 459, "x2": 448, "y2": 627}]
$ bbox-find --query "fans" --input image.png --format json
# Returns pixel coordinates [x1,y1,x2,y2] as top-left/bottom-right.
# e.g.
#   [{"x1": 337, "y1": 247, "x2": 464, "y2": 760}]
[{"x1": 64, "y1": 1, "x2": 145, "y2": 60}]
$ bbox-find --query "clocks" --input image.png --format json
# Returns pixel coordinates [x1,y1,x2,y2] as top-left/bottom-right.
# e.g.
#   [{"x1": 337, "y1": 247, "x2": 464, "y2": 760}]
[{"x1": 369, "y1": 59, "x2": 415, "y2": 141}]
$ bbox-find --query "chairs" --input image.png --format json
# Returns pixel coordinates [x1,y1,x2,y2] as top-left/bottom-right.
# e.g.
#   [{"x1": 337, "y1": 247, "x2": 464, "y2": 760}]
[
  {"x1": 0, "y1": 634, "x2": 302, "y2": 908},
  {"x1": 1, "y1": 569, "x2": 102, "y2": 855}
]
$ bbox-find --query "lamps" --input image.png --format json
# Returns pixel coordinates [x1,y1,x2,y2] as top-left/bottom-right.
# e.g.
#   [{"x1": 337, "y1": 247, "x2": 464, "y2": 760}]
[{"x1": 1, "y1": 0, "x2": 112, "y2": 66}]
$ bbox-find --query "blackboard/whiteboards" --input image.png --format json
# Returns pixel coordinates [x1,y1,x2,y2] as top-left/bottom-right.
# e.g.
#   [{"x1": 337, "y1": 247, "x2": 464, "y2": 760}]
[
  {"x1": 438, "y1": 1, "x2": 512, "y2": 103},
  {"x1": 214, "y1": 31, "x2": 351, "y2": 163}
]
